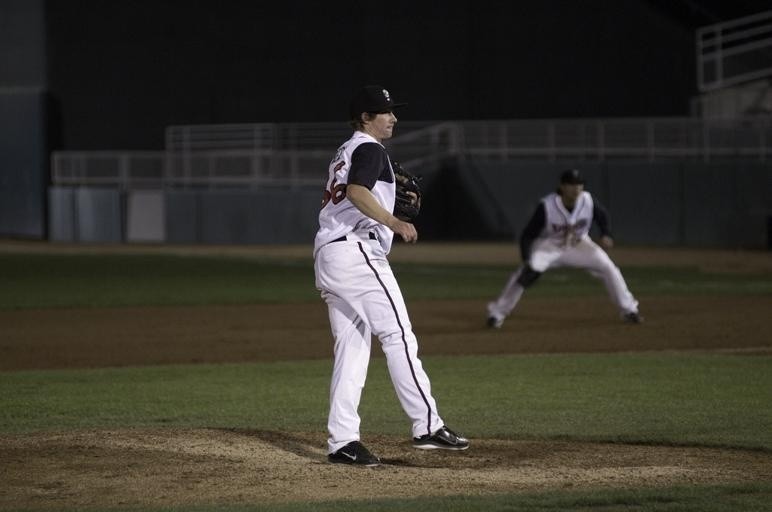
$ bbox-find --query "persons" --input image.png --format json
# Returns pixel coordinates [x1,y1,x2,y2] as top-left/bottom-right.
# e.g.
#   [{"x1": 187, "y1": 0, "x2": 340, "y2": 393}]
[
  {"x1": 487, "y1": 168, "x2": 645, "y2": 330},
  {"x1": 313, "y1": 83, "x2": 470, "y2": 466}
]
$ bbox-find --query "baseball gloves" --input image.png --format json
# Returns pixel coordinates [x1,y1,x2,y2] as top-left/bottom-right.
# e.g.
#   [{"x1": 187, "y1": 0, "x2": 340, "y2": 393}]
[{"x1": 392, "y1": 161, "x2": 423, "y2": 220}]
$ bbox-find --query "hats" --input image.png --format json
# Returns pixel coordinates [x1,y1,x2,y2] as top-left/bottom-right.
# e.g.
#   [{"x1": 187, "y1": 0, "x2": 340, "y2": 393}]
[
  {"x1": 351, "y1": 85, "x2": 406, "y2": 116},
  {"x1": 560, "y1": 168, "x2": 590, "y2": 185}
]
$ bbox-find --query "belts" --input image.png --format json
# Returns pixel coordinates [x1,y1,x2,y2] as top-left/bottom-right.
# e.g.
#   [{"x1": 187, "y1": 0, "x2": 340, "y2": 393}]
[{"x1": 330, "y1": 231, "x2": 377, "y2": 244}]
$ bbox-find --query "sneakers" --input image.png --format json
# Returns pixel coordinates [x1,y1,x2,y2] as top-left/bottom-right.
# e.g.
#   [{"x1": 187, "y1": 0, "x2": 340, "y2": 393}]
[
  {"x1": 327, "y1": 441, "x2": 381, "y2": 467},
  {"x1": 412, "y1": 426, "x2": 470, "y2": 452},
  {"x1": 486, "y1": 315, "x2": 503, "y2": 328},
  {"x1": 625, "y1": 313, "x2": 643, "y2": 323}
]
